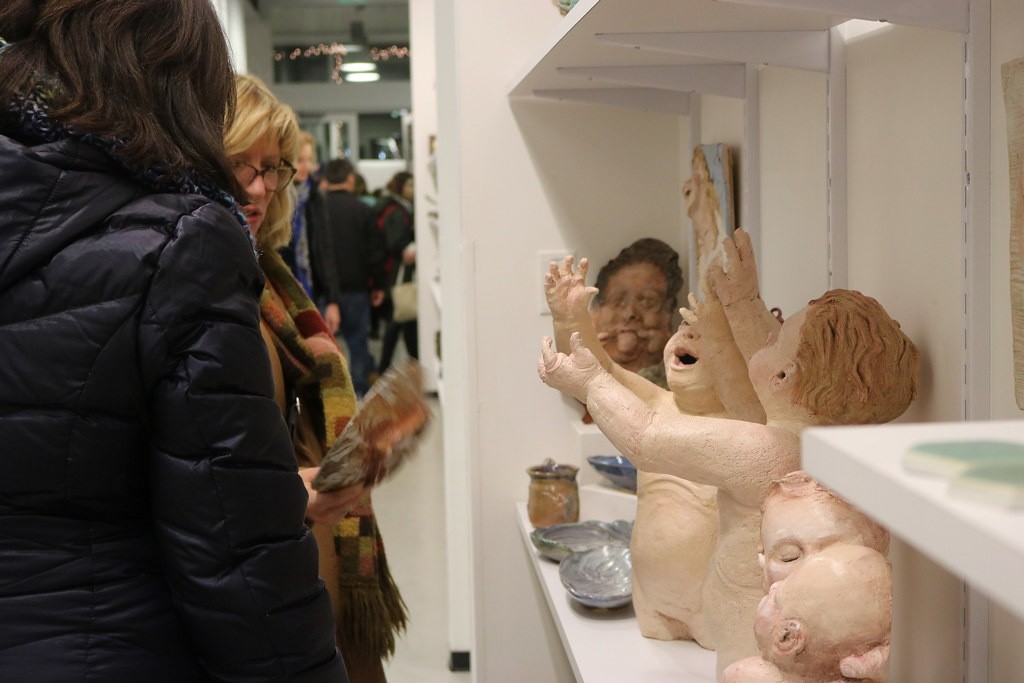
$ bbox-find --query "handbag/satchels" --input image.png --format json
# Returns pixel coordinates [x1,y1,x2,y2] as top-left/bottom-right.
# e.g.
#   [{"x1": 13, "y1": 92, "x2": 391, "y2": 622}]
[{"x1": 391, "y1": 263, "x2": 416, "y2": 323}]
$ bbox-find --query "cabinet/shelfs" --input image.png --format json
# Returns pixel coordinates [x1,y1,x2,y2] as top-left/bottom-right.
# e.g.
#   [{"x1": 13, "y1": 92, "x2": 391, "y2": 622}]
[{"x1": 450, "y1": 0, "x2": 1023, "y2": 683}]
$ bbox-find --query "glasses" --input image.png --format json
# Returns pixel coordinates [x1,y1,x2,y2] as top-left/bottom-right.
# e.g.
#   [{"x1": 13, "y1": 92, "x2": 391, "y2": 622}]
[{"x1": 232, "y1": 158, "x2": 297, "y2": 192}]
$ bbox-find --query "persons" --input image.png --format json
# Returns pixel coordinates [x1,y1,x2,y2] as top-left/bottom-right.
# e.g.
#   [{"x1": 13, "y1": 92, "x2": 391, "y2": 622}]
[
  {"x1": 221, "y1": 68, "x2": 385, "y2": 683},
  {"x1": 536, "y1": 231, "x2": 921, "y2": 682},
  {"x1": 0, "y1": 0, "x2": 349, "y2": 683},
  {"x1": 283, "y1": 133, "x2": 419, "y2": 398}
]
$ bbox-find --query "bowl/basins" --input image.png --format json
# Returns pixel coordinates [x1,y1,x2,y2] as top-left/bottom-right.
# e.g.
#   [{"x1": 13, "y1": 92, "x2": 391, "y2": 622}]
[{"x1": 588, "y1": 456, "x2": 638, "y2": 493}]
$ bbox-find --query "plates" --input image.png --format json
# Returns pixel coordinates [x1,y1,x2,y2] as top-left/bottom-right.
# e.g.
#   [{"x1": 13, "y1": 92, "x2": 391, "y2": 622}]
[{"x1": 530, "y1": 520, "x2": 633, "y2": 609}]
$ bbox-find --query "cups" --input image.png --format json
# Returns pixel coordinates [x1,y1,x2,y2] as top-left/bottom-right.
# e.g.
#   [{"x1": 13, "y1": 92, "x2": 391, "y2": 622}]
[{"x1": 528, "y1": 465, "x2": 580, "y2": 527}]
